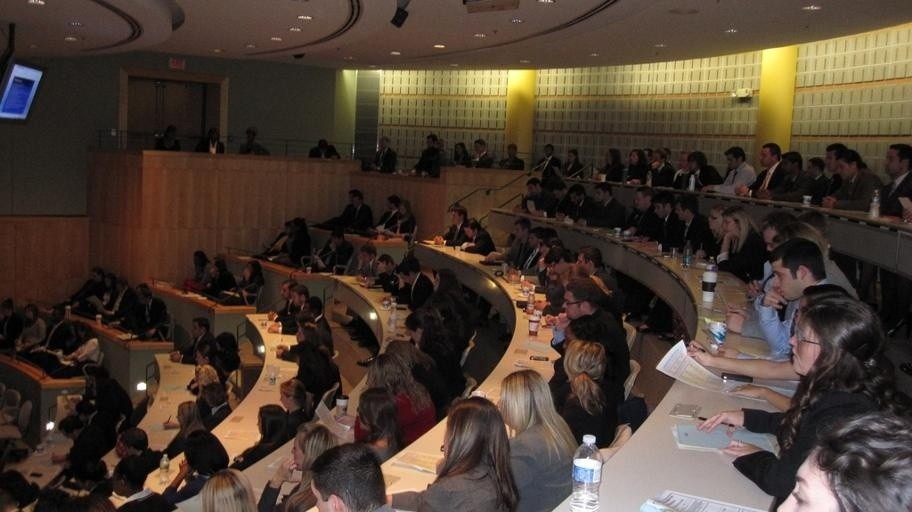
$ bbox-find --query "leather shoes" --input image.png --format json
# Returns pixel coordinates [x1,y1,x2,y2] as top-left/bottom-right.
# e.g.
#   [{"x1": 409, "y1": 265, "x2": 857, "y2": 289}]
[
  {"x1": 351, "y1": 335, "x2": 360, "y2": 340},
  {"x1": 356, "y1": 356, "x2": 376, "y2": 366}
]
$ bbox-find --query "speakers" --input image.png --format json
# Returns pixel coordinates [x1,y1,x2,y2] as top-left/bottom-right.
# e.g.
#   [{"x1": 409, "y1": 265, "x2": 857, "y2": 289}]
[{"x1": 391, "y1": 9, "x2": 408, "y2": 28}]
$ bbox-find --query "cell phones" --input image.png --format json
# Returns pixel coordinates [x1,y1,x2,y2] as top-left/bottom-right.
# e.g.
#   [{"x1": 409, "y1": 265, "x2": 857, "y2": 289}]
[
  {"x1": 722, "y1": 371, "x2": 753, "y2": 383},
  {"x1": 529, "y1": 355, "x2": 549, "y2": 361},
  {"x1": 32, "y1": 472, "x2": 41, "y2": 476}
]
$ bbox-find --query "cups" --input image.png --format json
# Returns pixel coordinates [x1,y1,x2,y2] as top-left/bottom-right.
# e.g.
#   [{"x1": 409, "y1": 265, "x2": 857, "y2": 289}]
[
  {"x1": 624, "y1": 231, "x2": 632, "y2": 239},
  {"x1": 701, "y1": 271, "x2": 718, "y2": 303},
  {"x1": 61, "y1": 390, "x2": 67, "y2": 402},
  {"x1": 383, "y1": 301, "x2": 389, "y2": 309},
  {"x1": 335, "y1": 395, "x2": 349, "y2": 418},
  {"x1": 803, "y1": 194, "x2": 813, "y2": 207},
  {"x1": 36, "y1": 445, "x2": 44, "y2": 454},
  {"x1": 614, "y1": 227, "x2": 622, "y2": 237},
  {"x1": 501, "y1": 264, "x2": 508, "y2": 275},
  {"x1": 95, "y1": 314, "x2": 101, "y2": 324},
  {"x1": 306, "y1": 266, "x2": 313, "y2": 275},
  {"x1": 599, "y1": 174, "x2": 607, "y2": 182},
  {"x1": 529, "y1": 316, "x2": 540, "y2": 336},
  {"x1": 708, "y1": 321, "x2": 728, "y2": 350},
  {"x1": 471, "y1": 390, "x2": 486, "y2": 398},
  {"x1": 671, "y1": 247, "x2": 680, "y2": 263}
]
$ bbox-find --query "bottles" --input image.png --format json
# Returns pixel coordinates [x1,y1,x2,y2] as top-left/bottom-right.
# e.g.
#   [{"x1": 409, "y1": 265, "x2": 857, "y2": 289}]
[
  {"x1": 706, "y1": 256, "x2": 716, "y2": 272},
  {"x1": 159, "y1": 454, "x2": 169, "y2": 484},
  {"x1": 647, "y1": 170, "x2": 653, "y2": 188},
  {"x1": 683, "y1": 241, "x2": 693, "y2": 267},
  {"x1": 269, "y1": 370, "x2": 277, "y2": 385},
  {"x1": 688, "y1": 175, "x2": 696, "y2": 192},
  {"x1": 387, "y1": 302, "x2": 397, "y2": 333},
  {"x1": 868, "y1": 189, "x2": 881, "y2": 217},
  {"x1": 526, "y1": 291, "x2": 536, "y2": 315},
  {"x1": 623, "y1": 168, "x2": 629, "y2": 184},
  {"x1": 65, "y1": 306, "x2": 71, "y2": 320},
  {"x1": 571, "y1": 435, "x2": 603, "y2": 512}
]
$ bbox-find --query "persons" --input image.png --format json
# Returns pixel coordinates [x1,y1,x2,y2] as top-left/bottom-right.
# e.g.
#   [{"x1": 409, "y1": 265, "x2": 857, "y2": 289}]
[
  {"x1": 154, "y1": 126, "x2": 181, "y2": 151},
  {"x1": 777, "y1": 411, "x2": 912, "y2": 512},
  {"x1": 412, "y1": 133, "x2": 442, "y2": 178},
  {"x1": 696, "y1": 283, "x2": 907, "y2": 503},
  {"x1": 240, "y1": 128, "x2": 270, "y2": 156},
  {"x1": 309, "y1": 139, "x2": 341, "y2": 159},
  {"x1": 522, "y1": 142, "x2": 912, "y2": 357},
  {"x1": 0, "y1": 188, "x2": 645, "y2": 512},
  {"x1": 452, "y1": 140, "x2": 525, "y2": 170},
  {"x1": 195, "y1": 128, "x2": 226, "y2": 154},
  {"x1": 369, "y1": 138, "x2": 397, "y2": 174}
]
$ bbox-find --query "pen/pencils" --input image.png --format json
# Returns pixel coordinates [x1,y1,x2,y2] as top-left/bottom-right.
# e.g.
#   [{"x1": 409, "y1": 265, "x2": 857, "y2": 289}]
[
  {"x1": 693, "y1": 345, "x2": 705, "y2": 353},
  {"x1": 167, "y1": 415, "x2": 172, "y2": 423},
  {"x1": 698, "y1": 416, "x2": 736, "y2": 427}
]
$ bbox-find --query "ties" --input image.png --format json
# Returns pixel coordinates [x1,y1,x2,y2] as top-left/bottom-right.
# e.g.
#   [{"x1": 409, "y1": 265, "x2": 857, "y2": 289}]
[
  {"x1": 524, "y1": 248, "x2": 537, "y2": 269},
  {"x1": 761, "y1": 173, "x2": 771, "y2": 190},
  {"x1": 731, "y1": 173, "x2": 738, "y2": 186}
]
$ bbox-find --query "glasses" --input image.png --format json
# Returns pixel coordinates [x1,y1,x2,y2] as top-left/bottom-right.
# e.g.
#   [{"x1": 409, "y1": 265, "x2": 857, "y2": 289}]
[{"x1": 794, "y1": 328, "x2": 819, "y2": 346}]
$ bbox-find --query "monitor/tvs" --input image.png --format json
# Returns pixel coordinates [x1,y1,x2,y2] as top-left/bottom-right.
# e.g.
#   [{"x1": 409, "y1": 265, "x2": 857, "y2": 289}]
[{"x1": 1, "y1": 57, "x2": 48, "y2": 125}]
